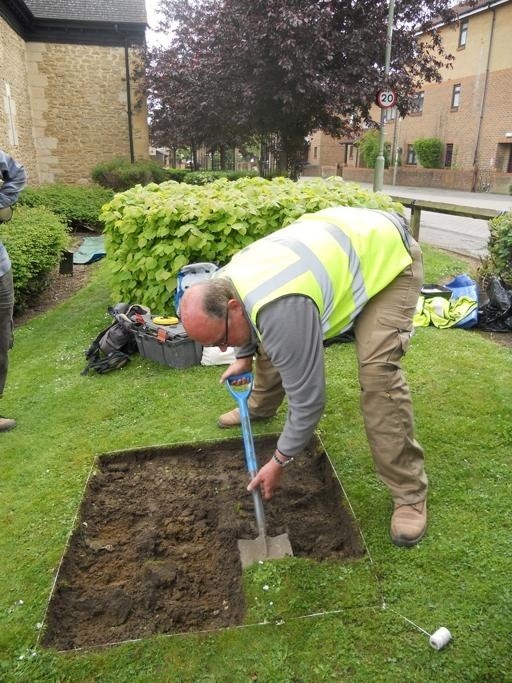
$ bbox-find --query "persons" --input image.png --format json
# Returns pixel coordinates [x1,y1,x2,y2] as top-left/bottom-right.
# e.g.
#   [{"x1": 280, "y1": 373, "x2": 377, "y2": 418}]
[
  {"x1": 178, "y1": 203, "x2": 427, "y2": 549},
  {"x1": 1, "y1": 151, "x2": 28, "y2": 432}
]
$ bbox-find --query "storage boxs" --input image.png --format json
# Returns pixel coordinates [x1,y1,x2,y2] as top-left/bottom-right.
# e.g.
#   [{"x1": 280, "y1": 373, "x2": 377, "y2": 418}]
[{"x1": 129, "y1": 314, "x2": 204, "y2": 369}]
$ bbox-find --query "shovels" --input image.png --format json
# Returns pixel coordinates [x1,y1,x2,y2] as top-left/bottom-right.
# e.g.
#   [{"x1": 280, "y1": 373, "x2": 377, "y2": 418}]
[{"x1": 225, "y1": 372, "x2": 294, "y2": 572}]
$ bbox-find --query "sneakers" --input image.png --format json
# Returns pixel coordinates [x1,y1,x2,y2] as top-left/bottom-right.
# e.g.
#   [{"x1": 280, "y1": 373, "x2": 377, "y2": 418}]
[
  {"x1": 389, "y1": 499, "x2": 427, "y2": 547},
  {"x1": 0, "y1": 417, "x2": 16, "y2": 432},
  {"x1": 217, "y1": 406, "x2": 255, "y2": 428}
]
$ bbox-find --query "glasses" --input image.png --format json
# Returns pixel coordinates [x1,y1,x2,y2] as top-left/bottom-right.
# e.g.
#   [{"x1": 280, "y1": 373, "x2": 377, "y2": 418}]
[{"x1": 213, "y1": 302, "x2": 229, "y2": 347}]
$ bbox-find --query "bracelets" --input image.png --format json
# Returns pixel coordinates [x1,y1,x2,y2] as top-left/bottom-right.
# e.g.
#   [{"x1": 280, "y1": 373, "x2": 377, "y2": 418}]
[{"x1": 271, "y1": 453, "x2": 295, "y2": 468}]
